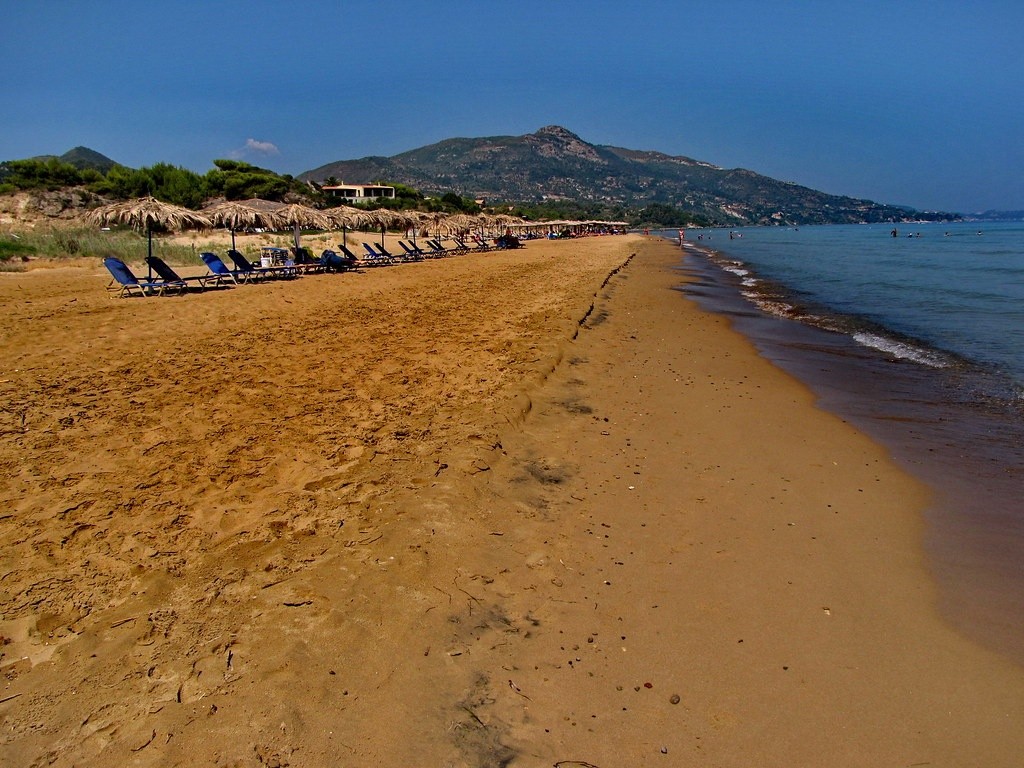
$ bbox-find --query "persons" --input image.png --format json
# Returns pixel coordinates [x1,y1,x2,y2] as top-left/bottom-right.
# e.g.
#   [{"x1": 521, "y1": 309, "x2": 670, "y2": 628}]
[
  {"x1": 944, "y1": 232, "x2": 950, "y2": 237},
  {"x1": 679, "y1": 228, "x2": 685, "y2": 246},
  {"x1": 978, "y1": 231, "x2": 982, "y2": 235},
  {"x1": 506, "y1": 226, "x2": 511, "y2": 236},
  {"x1": 730, "y1": 232, "x2": 735, "y2": 239},
  {"x1": 908, "y1": 232, "x2": 920, "y2": 238},
  {"x1": 891, "y1": 228, "x2": 896, "y2": 237},
  {"x1": 543, "y1": 228, "x2": 546, "y2": 239},
  {"x1": 737, "y1": 233, "x2": 743, "y2": 238},
  {"x1": 643, "y1": 226, "x2": 649, "y2": 235},
  {"x1": 698, "y1": 235, "x2": 704, "y2": 240}
]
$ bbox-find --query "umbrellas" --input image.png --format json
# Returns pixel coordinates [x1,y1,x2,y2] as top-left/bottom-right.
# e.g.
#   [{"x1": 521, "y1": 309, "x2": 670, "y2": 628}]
[
  {"x1": 193, "y1": 199, "x2": 272, "y2": 284},
  {"x1": 268, "y1": 202, "x2": 536, "y2": 253},
  {"x1": 77, "y1": 195, "x2": 212, "y2": 295},
  {"x1": 537, "y1": 220, "x2": 630, "y2": 234}
]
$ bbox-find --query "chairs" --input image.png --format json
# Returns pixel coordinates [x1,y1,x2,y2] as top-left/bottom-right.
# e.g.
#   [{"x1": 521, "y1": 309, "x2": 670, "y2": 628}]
[
  {"x1": 408, "y1": 240, "x2": 437, "y2": 259},
  {"x1": 362, "y1": 242, "x2": 392, "y2": 264},
  {"x1": 199, "y1": 252, "x2": 268, "y2": 285},
  {"x1": 373, "y1": 242, "x2": 405, "y2": 264},
  {"x1": 398, "y1": 241, "x2": 424, "y2": 260},
  {"x1": 144, "y1": 256, "x2": 223, "y2": 294},
  {"x1": 226, "y1": 250, "x2": 278, "y2": 281},
  {"x1": 426, "y1": 234, "x2": 489, "y2": 259},
  {"x1": 102, "y1": 257, "x2": 180, "y2": 299}
]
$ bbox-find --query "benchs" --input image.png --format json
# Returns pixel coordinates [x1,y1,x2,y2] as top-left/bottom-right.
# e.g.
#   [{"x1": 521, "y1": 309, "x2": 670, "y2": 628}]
[{"x1": 254, "y1": 264, "x2": 320, "y2": 279}]
